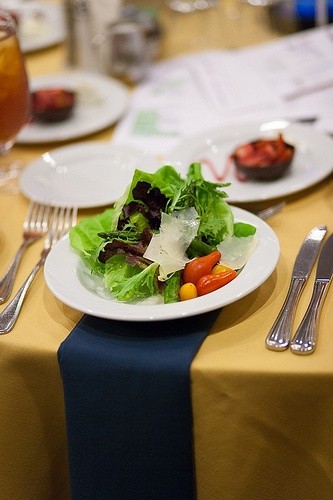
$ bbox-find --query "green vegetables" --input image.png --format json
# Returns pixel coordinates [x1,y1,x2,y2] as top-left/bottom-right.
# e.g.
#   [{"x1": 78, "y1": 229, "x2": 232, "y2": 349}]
[{"x1": 69, "y1": 162, "x2": 256, "y2": 303}]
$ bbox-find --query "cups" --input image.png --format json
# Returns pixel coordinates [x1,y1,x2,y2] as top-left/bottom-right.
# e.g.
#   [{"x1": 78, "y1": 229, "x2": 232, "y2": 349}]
[{"x1": 98, "y1": 20, "x2": 148, "y2": 84}]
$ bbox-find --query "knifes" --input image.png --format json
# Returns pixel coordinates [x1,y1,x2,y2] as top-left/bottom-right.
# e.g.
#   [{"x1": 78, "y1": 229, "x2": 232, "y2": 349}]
[
  {"x1": 290, "y1": 234, "x2": 333, "y2": 356},
  {"x1": 265, "y1": 225, "x2": 327, "y2": 352}
]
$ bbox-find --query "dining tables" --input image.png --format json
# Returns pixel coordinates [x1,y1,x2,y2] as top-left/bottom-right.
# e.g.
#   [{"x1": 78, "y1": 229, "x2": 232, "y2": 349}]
[{"x1": 1, "y1": 1, "x2": 333, "y2": 500}]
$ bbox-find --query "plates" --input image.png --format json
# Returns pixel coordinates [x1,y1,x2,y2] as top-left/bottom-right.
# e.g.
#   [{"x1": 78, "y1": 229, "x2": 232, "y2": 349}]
[
  {"x1": 19, "y1": 142, "x2": 139, "y2": 208},
  {"x1": 44, "y1": 204, "x2": 280, "y2": 321},
  {"x1": 14, "y1": 71, "x2": 130, "y2": 143},
  {"x1": 0, "y1": 0, "x2": 67, "y2": 54},
  {"x1": 179, "y1": 122, "x2": 333, "y2": 203}
]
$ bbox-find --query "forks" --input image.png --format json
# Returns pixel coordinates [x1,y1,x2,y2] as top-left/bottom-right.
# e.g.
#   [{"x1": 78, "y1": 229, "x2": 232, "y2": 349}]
[
  {"x1": 0, "y1": 199, "x2": 53, "y2": 305},
  {"x1": 0, "y1": 202, "x2": 78, "y2": 335}
]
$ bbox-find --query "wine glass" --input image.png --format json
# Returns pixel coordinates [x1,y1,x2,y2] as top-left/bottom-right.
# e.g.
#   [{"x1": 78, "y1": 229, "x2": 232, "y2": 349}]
[{"x1": 0, "y1": 13, "x2": 30, "y2": 188}]
